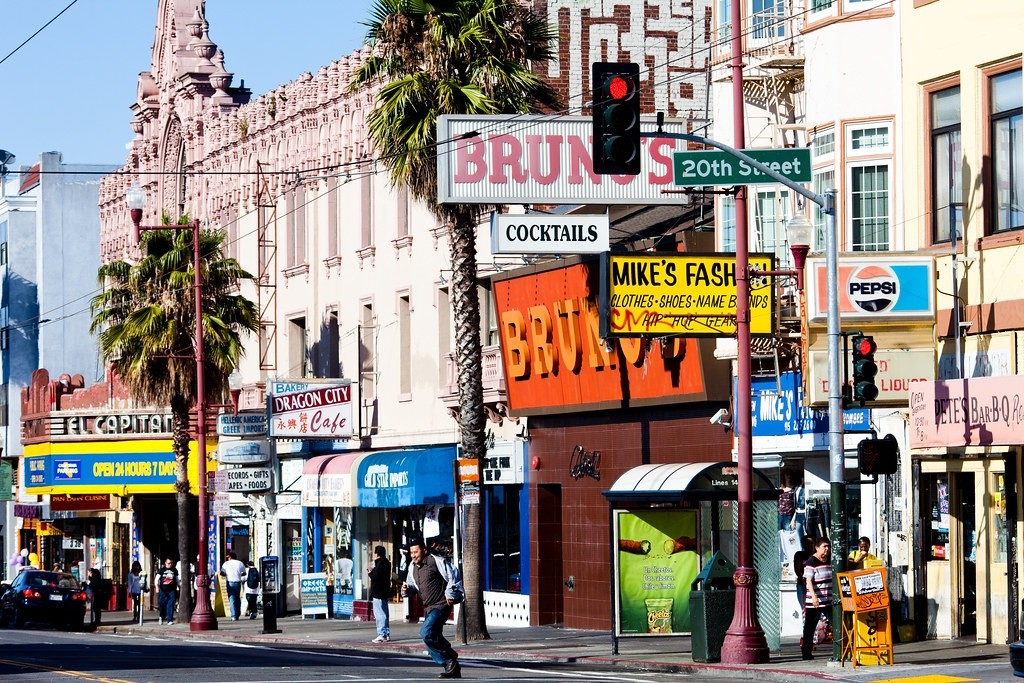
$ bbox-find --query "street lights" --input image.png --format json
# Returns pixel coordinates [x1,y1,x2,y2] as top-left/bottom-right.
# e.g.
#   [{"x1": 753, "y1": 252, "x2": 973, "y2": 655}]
[{"x1": 125, "y1": 179, "x2": 219, "y2": 631}]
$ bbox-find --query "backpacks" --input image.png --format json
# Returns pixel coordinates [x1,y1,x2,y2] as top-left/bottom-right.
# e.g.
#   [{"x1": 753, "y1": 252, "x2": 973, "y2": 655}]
[{"x1": 245, "y1": 567, "x2": 259, "y2": 589}]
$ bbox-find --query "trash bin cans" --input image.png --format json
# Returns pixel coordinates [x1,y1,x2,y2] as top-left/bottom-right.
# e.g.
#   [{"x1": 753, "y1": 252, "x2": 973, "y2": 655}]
[{"x1": 688, "y1": 549, "x2": 739, "y2": 663}]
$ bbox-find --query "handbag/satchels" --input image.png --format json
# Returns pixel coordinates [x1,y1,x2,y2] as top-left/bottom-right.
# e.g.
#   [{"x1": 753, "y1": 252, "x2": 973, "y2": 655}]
[{"x1": 142, "y1": 584, "x2": 150, "y2": 593}]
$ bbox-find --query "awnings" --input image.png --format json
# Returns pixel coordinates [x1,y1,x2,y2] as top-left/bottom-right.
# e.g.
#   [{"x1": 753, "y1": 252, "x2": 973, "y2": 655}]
[{"x1": 301, "y1": 447, "x2": 454, "y2": 507}]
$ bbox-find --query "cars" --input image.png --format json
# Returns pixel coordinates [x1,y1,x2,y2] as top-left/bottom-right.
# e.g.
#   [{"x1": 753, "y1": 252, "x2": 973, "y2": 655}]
[{"x1": 0, "y1": 570, "x2": 87, "y2": 628}]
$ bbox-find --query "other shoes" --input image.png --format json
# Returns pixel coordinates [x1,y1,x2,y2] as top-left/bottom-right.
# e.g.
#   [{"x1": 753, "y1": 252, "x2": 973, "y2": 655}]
[
  {"x1": 230, "y1": 616, "x2": 240, "y2": 622},
  {"x1": 158, "y1": 617, "x2": 163, "y2": 625},
  {"x1": 802, "y1": 654, "x2": 815, "y2": 660},
  {"x1": 166, "y1": 621, "x2": 174, "y2": 625},
  {"x1": 250, "y1": 612, "x2": 258, "y2": 620}
]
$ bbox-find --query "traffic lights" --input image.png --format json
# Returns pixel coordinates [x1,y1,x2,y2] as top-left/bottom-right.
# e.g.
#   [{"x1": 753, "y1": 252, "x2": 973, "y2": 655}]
[
  {"x1": 859, "y1": 438, "x2": 898, "y2": 476},
  {"x1": 591, "y1": 61, "x2": 641, "y2": 176},
  {"x1": 852, "y1": 336, "x2": 880, "y2": 402}
]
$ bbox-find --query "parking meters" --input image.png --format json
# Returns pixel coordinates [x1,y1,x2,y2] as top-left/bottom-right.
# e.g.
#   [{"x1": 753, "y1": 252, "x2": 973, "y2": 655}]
[{"x1": 137, "y1": 572, "x2": 148, "y2": 626}]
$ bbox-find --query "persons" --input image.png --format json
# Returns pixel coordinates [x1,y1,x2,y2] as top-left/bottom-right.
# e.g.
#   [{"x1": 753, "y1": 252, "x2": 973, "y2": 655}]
[
  {"x1": 71, "y1": 561, "x2": 80, "y2": 583},
  {"x1": 155, "y1": 558, "x2": 180, "y2": 625},
  {"x1": 176, "y1": 560, "x2": 193, "y2": 585},
  {"x1": 794, "y1": 536, "x2": 816, "y2": 651},
  {"x1": 802, "y1": 538, "x2": 832, "y2": 659},
  {"x1": 128, "y1": 561, "x2": 149, "y2": 622},
  {"x1": 82, "y1": 582, "x2": 94, "y2": 624},
  {"x1": 47, "y1": 563, "x2": 60, "y2": 585},
  {"x1": 88, "y1": 568, "x2": 102, "y2": 625},
  {"x1": 848, "y1": 537, "x2": 880, "y2": 569},
  {"x1": 240, "y1": 561, "x2": 260, "y2": 619},
  {"x1": 401, "y1": 540, "x2": 464, "y2": 677},
  {"x1": 220, "y1": 552, "x2": 246, "y2": 621},
  {"x1": 367, "y1": 546, "x2": 391, "y2": 643}
]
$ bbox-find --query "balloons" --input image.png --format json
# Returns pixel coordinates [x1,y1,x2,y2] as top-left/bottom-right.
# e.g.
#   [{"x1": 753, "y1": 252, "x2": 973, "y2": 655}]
[{"x1": 10, "y1": 548, "x2": 41, "y2": 567}]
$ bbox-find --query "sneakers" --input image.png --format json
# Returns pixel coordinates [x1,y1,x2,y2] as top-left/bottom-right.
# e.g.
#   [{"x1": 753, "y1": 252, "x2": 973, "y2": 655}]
[
  {"x1": 372, "y1": 635, "x2": 391, "y2": 643},
  {"x1": 438, "y1": 653, "x2": 462, "y2": 678}
]
